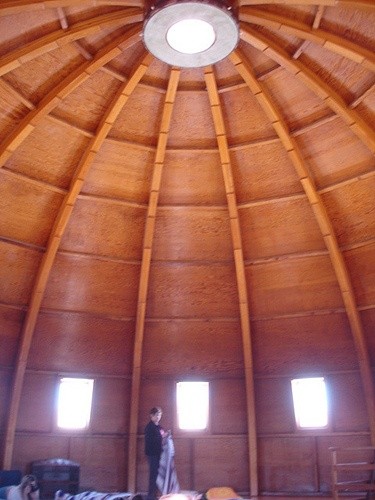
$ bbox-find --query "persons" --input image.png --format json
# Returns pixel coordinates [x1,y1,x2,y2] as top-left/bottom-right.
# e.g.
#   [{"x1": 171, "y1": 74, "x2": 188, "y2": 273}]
[{"x1": 144, "y1": 407, "x2": 168, "y2": 500}]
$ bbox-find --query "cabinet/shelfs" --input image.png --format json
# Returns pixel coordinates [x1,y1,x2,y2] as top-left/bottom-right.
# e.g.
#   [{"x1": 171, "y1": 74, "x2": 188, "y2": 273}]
[{"x1": 30, "y1": 459, "x2": 80, "y2": 500}]
